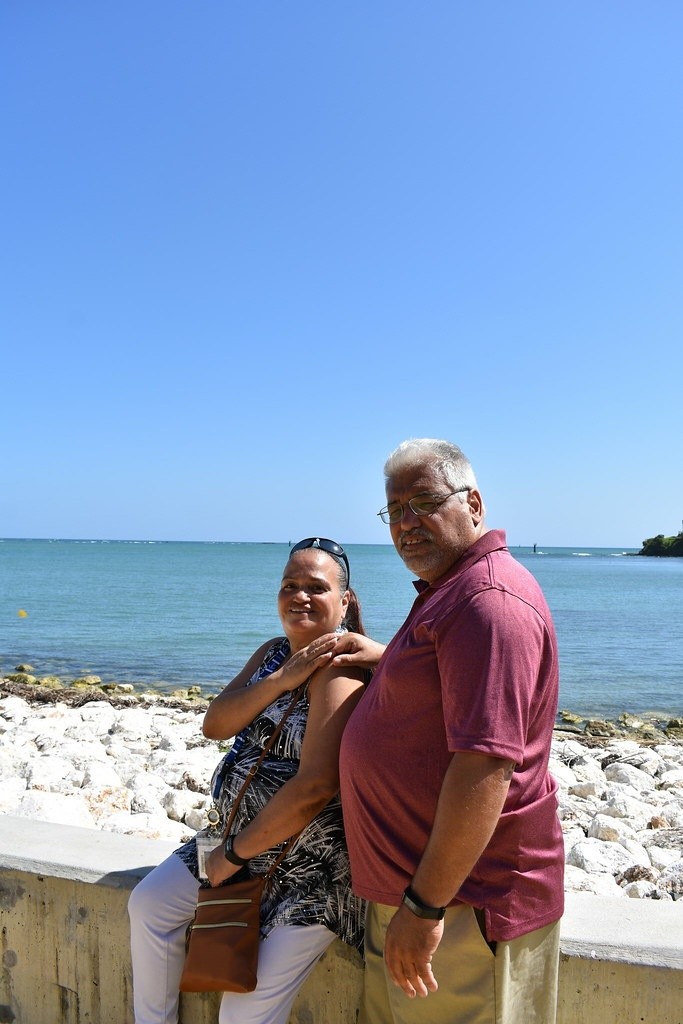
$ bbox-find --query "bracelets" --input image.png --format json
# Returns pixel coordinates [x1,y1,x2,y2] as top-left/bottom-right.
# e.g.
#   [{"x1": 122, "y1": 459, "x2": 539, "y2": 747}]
[{"x1": 402, "y1": 886, "x2": 445, "y2": 920}]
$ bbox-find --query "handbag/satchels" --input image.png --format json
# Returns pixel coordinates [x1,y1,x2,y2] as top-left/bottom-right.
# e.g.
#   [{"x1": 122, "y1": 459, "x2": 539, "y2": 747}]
[{"x1": 179, "y1": 874, "x2": 265, "y2": 993}]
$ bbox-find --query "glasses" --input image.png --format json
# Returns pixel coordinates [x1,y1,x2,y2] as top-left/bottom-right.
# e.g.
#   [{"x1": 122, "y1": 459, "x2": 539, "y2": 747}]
[
  {"x1": 289, "y1": 537, "x2": 350, "y2": 590},
  {"x1": 377, "y1": 489, "x2": 470, "y2": 524}
]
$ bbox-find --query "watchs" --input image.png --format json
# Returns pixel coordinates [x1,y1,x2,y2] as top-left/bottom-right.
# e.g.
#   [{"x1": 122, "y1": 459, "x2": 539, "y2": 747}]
[{"x1": 224, "y1": 835, "x2": 250, "y2": 866}]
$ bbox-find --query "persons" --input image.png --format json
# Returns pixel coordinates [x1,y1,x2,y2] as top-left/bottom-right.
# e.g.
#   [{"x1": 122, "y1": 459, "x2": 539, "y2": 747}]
[
  {"x1": 322, "y1": 439, "x2": 564, "y2": 1024},
  {"x1": 129, "y1": 542, "x2": 374, "y2": 1024}
]
